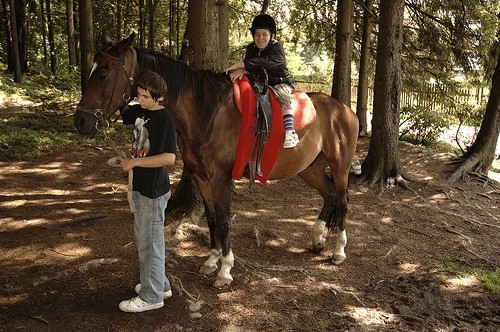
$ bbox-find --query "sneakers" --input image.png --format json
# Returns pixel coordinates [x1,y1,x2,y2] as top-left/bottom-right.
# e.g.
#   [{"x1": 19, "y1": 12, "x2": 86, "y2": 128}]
[
  {"x1": 135, "y1": 283, "x2": 172, "y2": 299},
  {"x1": 119, "y1": 296, "x2": 164, "y2": 312},
  {"x1": 283, "y1": 132, "x2": 299, "y2": 149}
]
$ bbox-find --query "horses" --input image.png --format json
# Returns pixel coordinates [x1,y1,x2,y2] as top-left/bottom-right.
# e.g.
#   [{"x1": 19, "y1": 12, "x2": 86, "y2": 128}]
[{"x1": 74, "y1": 31, "x2": 361, "y2": 289}]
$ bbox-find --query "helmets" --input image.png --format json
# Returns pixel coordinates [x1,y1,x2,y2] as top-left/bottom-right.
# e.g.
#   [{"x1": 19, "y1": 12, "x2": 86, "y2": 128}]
[{"x1": 249, "y1": 14, "x2": 276, "y2": 36}]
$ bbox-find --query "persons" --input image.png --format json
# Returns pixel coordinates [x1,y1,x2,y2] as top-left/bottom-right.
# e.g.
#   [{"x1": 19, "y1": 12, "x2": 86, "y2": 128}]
[
  {"x1": 222, "y1": 14, "x2": 300, "y2": 148},
  {"x1": 118, "y1": 71, "x2": 177, "y2": 312}
]
